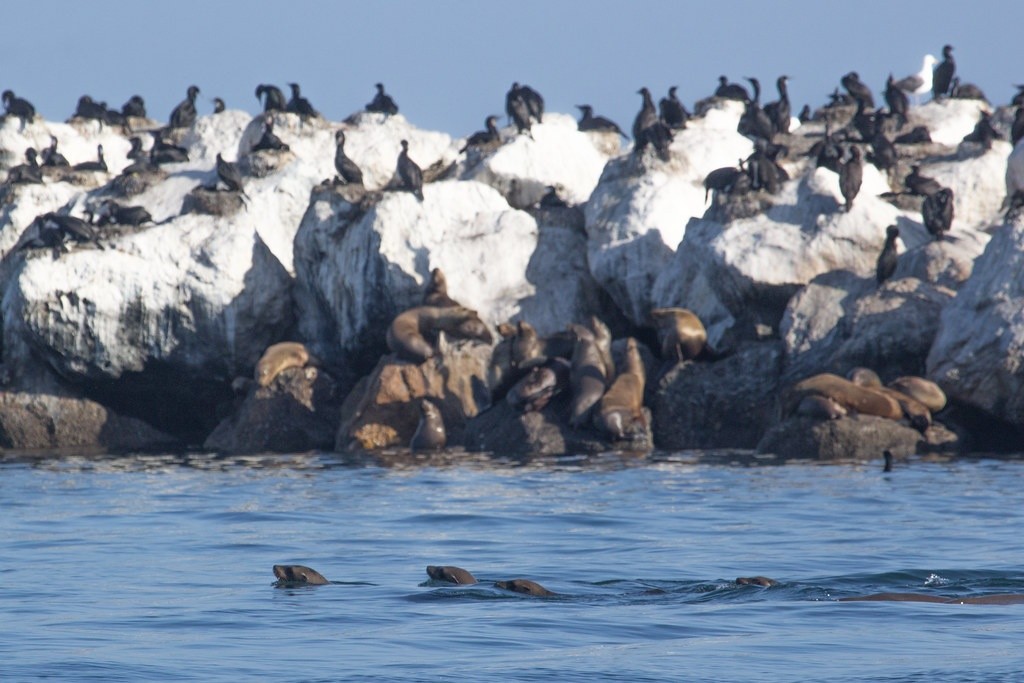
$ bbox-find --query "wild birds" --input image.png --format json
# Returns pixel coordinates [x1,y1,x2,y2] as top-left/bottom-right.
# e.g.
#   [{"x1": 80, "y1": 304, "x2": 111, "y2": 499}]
[
  {"x1": 216, "y1": 152, "x2": 251, "y2": 202},
  {"x1": 335, "y1": 131, "x2": 363, "y2": 185},
  {"x1": 577, "y1": 44, "x2": 1024, "y2": 287},
  {"x1": 397, "y1": 140, "x2": 425, "y2": 201},
  {"x1": 458, "y1": 82, "x2": 545, "y2": 155},
  {"x1": 3, "y1": 85, "x2": 224, "y2": 253},
  {"x1": 251, "y1": 83, "x2": 317, "y2": 151},
  {"x1": 365, "y1": 83, "x2": 398, "y2": 115}
]
